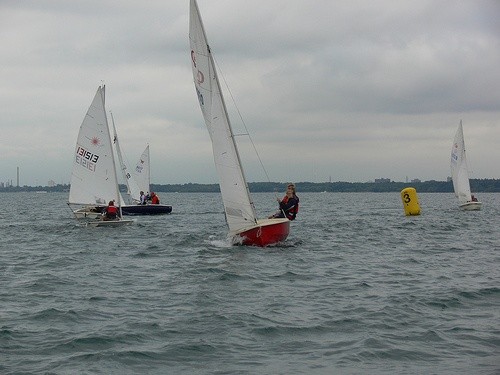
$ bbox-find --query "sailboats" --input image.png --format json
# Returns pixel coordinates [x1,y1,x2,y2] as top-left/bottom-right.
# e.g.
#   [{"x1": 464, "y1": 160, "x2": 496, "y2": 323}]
[
  {"x1": 188, "y1": 1, "x2": 289, "y2": 248},
  {"x1": 68, "y1": 84, "x2": 173, "y2": 227},
  {"x1": 449, "y1": 120, "x2": 482, "y2": 210}
]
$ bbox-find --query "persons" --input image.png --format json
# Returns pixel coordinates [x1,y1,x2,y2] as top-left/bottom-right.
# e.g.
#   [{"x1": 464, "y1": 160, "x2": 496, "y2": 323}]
[
  {"x1": 137, "y1": 191, "x2": 159, "y2": 205},
  {"x1": 102, "y1": 200, "x2": 121, "y2": 222},
  {"x1": 268, "y1": 184, "x2": 300, "y2": 221}
]
